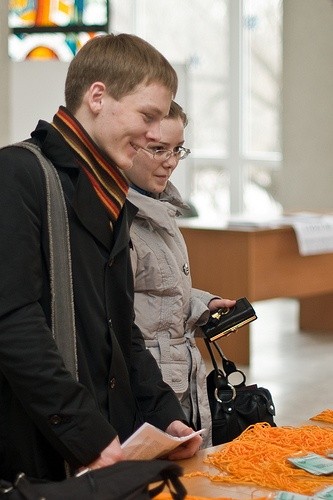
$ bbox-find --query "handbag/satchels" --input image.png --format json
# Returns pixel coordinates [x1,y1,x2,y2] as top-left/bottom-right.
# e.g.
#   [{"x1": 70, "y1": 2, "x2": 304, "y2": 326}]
[
  {"x1": 205, "y1": 335, "x2": 277, "y2": 444},
  {"x1": 12, "y1": 460, "x2": 187, "y2": 500}
]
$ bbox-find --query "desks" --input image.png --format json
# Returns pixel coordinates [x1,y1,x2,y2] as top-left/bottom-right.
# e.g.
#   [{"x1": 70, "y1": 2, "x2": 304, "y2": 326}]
[
  {"x1": 175, "y1": 212, "x2": 332, "y2": 367},
  {"x1": 149, "y1": 410, "x2": 333, "y2": 500}
]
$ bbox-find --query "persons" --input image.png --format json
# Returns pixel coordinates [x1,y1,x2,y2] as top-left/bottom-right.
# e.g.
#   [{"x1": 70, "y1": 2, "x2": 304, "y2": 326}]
[
  {"x1": 0, "y1": 33, "x2": 203, "y2": 500},
  {"x1": 128, "y1": 101, "x2": 235, "y2": 463}
]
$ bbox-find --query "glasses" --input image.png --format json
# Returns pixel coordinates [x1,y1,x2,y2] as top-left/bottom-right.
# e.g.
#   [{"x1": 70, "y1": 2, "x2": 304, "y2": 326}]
[{"x1": 140, "y1": 145, "x2": 192, "y2": 163}]
